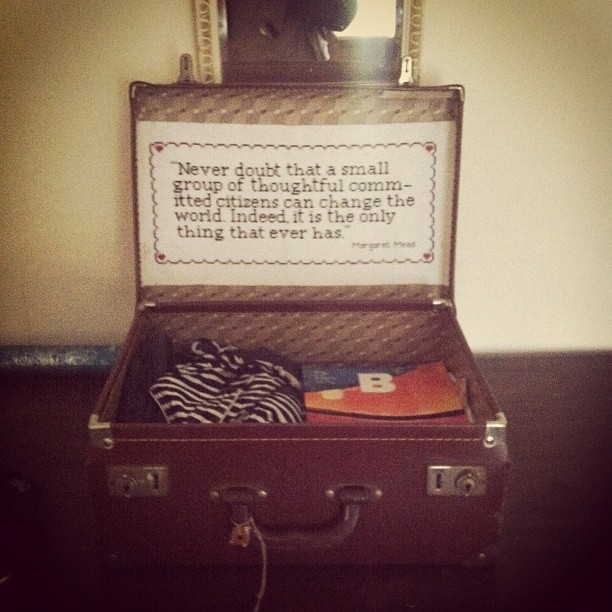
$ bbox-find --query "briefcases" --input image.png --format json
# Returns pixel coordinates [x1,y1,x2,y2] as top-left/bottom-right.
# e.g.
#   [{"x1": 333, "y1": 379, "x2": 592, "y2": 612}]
[{"x1": 87, "y1": 55, "x2": 510, "y2": 576}]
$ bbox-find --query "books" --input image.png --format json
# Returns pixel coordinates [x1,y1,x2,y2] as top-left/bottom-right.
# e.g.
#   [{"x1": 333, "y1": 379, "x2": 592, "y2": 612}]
[{"x1": 300, "y1": 360, "x2": 471, "y2": 428}]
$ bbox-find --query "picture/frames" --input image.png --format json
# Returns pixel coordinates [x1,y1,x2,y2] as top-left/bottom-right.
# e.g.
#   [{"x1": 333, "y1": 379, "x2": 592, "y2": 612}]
[{"x1": 193, "y1": 0, "x2": 423, "y2": 86}]
